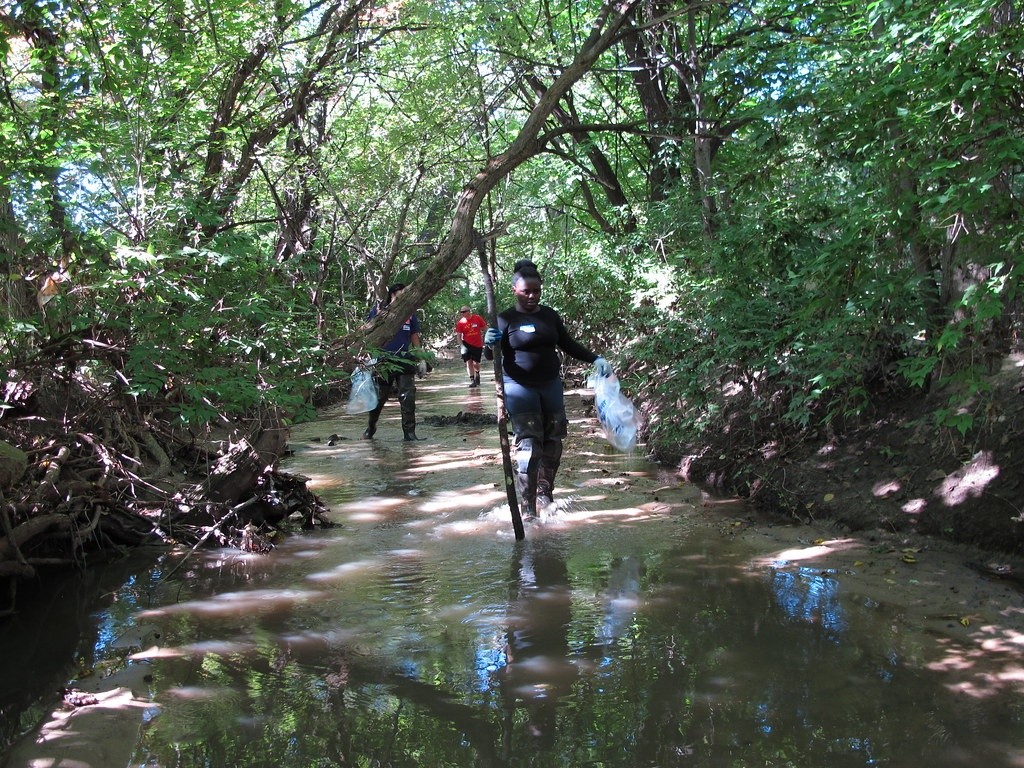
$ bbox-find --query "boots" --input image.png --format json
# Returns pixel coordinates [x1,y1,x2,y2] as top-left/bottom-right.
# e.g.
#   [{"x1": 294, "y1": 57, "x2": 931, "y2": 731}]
[
  {"x1": 402, "y1": 412, "x2": 427, "y2": 441},
  {"x1": 538, "y1": 466, "x2": 557, "y2": 509},
  {"x1": 517, "y1": 473, "x2": 537, "y2": 524},
  {"x1": 362, "y1": 412, "x2": 376, "y2": 438}
]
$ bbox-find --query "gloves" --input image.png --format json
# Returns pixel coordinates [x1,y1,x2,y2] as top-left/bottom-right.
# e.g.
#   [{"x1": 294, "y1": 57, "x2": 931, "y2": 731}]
[
  {"x1": 595, "y1": 355, "x2": 612, "y2": 378},
  {"x1": 418, "y1": 359, "x2": 427, "y2": 379},
  {"x1": 460, "y1": 343, "x2": 468, "y2": 354},
  {"x1": 484, "y1": 327, "x2": 502, "y2": 344}
]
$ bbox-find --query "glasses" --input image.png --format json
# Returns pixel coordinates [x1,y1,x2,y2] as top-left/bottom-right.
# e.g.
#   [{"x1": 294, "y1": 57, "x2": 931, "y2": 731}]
[{"x1": 460, "y1": 310, "x2": 470, "y2": 313}]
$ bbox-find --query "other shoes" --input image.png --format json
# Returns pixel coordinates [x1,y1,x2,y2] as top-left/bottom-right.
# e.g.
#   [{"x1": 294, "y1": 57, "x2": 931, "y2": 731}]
[
  {"x1": 469, "y1": 380, "x2": 476, "y2": 388},
  {"x1": 475, "y1": 372, "x2": 480, "y2": 385}
]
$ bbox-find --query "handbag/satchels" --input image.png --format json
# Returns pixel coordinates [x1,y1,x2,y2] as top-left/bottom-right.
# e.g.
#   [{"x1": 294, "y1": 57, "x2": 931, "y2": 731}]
[
  {"x1": 587, "y1": 370, "x2": 642, "y2": 453},
  {"x1": 347, "y1": 365, "x2": 378, "y2": 414}
]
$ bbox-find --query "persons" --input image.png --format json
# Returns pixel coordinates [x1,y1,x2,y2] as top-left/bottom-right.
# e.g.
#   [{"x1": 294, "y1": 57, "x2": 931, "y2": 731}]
[
  {"x1": 363, "y1": 283, "x2": 428, "y2": 441},
  {"x1": 456, "y1": 305, "x2": 490, "y2": 388},
  {"x1": 483, "y1": 259, "x2": 612, "y2": 525}
]
